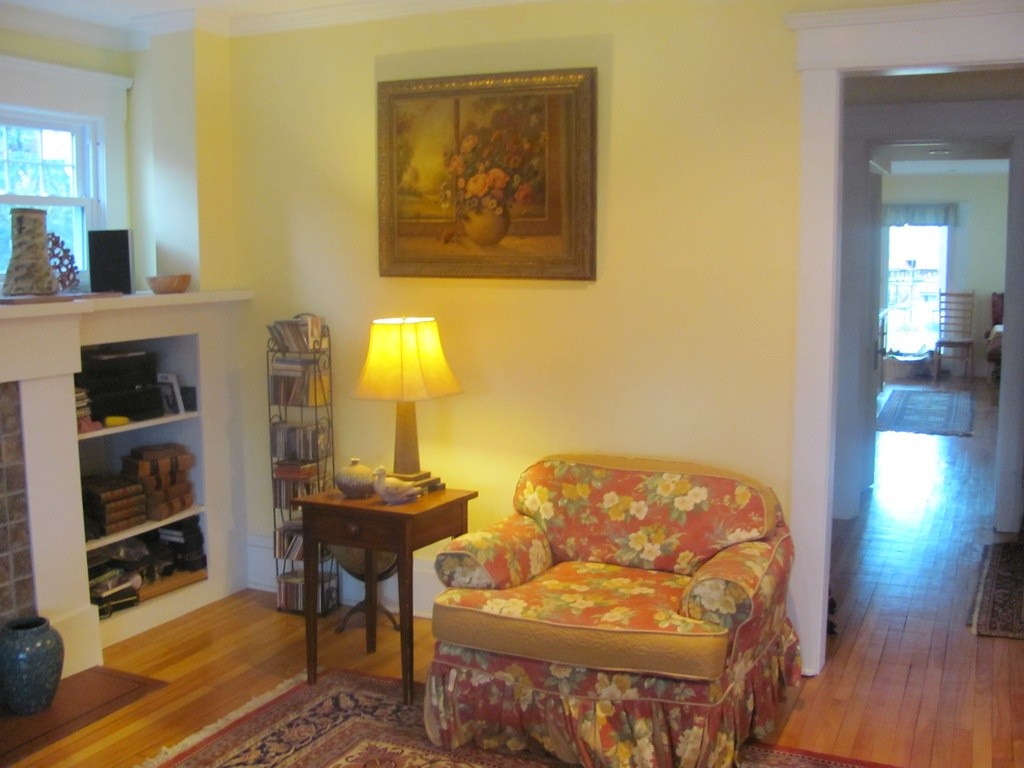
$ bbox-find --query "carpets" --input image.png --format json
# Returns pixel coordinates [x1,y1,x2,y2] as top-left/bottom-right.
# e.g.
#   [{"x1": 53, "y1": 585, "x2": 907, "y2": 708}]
[
  {"x1": 876, "y1": 390, "x2": 975, "y2": 436},
  {"x1": 151, "y1": 670, "x2": 890, "y2": 768},
  {"x1": 966, "y1": 540, "x2": 1024, "y2": 639}
]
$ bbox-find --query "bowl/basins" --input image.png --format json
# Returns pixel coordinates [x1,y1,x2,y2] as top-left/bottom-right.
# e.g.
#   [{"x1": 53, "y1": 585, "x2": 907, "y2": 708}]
[{"x1": 146, "y1": 274, "x2": 191, "y2": 294}]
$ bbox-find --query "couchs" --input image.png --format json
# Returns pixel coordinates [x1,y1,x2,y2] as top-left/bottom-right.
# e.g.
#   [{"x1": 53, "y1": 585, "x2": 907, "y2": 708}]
[{"x1": 424, "y1": 457, "x2": 801, "y2": 768}]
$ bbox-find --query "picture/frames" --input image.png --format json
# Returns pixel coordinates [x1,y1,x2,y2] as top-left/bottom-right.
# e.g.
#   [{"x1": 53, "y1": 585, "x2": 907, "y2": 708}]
[{"x1": 379, "y1": 63, "x2": 595, "y2": 283}]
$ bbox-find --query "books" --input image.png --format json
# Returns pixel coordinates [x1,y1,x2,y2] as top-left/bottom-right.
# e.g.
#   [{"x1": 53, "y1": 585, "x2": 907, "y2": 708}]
[
  {"x1": 88, "y1": 349, "x2": 144, "y2": 360},
  {"x1": 272, "y1": 423, "x2": 331, "y2": 458},
  {"x1": 75, "y1": 389, "x2": 91, "y2": 418},
  {"x1": 87, "y1": 475, "x2": 144, "y2": 504},
  {"x1": 88, "y1": 568, "x2": 137, "y2": 615},
  {"x1": 276, "y1": 518, "x2": 332, "y2": 560},
  {"x1": 274, "y1": 460, "x2": 334, "y2": 510},
  {"x1": 275, "y1": 570, "x2": 338, "y2": 614},
  {"x1": 267, "y1": 317, "x2": 320, "y2": 354},
  {"x1": 85, "y1": 444, "x2": 194, "y2": 536},
  {"x1": 158, "y1": 372, "x2": 185, "y2": 413},
  {"x1": 88, "y1": 229, "x2": 136, "y2": 295},
  {"x1": 268, "y1": 359, "x2": 330, "y2": 405}
]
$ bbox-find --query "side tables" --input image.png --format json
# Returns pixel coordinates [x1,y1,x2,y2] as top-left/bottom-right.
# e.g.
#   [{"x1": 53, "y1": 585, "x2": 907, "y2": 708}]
[{"x1": 294, "y1": 489, "x2": 478, "y2": 705}]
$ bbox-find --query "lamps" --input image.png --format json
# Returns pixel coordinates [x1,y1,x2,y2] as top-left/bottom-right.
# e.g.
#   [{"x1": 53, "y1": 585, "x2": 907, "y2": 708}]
[{"x1": 354, "y1": 317, "x2": 461, "y2": 496}]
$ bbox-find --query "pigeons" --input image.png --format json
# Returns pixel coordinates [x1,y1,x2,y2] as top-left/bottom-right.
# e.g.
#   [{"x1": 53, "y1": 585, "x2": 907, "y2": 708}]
[{"x1": 370, "y1": 466, "x2": 424, "y2": 505}]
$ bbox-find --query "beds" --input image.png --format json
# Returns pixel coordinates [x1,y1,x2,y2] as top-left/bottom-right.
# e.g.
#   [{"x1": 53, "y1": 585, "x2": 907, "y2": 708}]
[{"x1": 984, "y1": 293, "x2": 1004, "y2": 405}]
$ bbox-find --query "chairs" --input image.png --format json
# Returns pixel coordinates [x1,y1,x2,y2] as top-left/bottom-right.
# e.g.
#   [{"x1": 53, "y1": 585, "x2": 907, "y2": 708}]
[{"x1": 934, "y1": 287, "x2": 976, "y2": 382}]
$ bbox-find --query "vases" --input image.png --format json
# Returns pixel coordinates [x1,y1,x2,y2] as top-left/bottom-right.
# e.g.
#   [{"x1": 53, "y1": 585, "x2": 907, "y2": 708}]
[{"x1": 0, "y1": 616, "x2": 64, "y2": 716}]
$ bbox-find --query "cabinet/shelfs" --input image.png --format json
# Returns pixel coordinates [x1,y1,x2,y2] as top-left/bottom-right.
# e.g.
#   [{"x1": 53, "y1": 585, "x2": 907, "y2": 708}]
[
  {"x1": 265, "y1": 323, "x2": 339, "y2": 617},
  {"x1": 74, "y1": 411, "x2": 207, "y2": 552},
  {"x1": 875, "y1": 316, "x2": 888, "y2": 395}
]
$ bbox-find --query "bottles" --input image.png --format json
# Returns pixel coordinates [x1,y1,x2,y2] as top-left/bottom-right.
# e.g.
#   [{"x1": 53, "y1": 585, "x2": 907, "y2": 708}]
[{"x1": 335, "y1": 458, "x2": 377, "y2": 498}]
